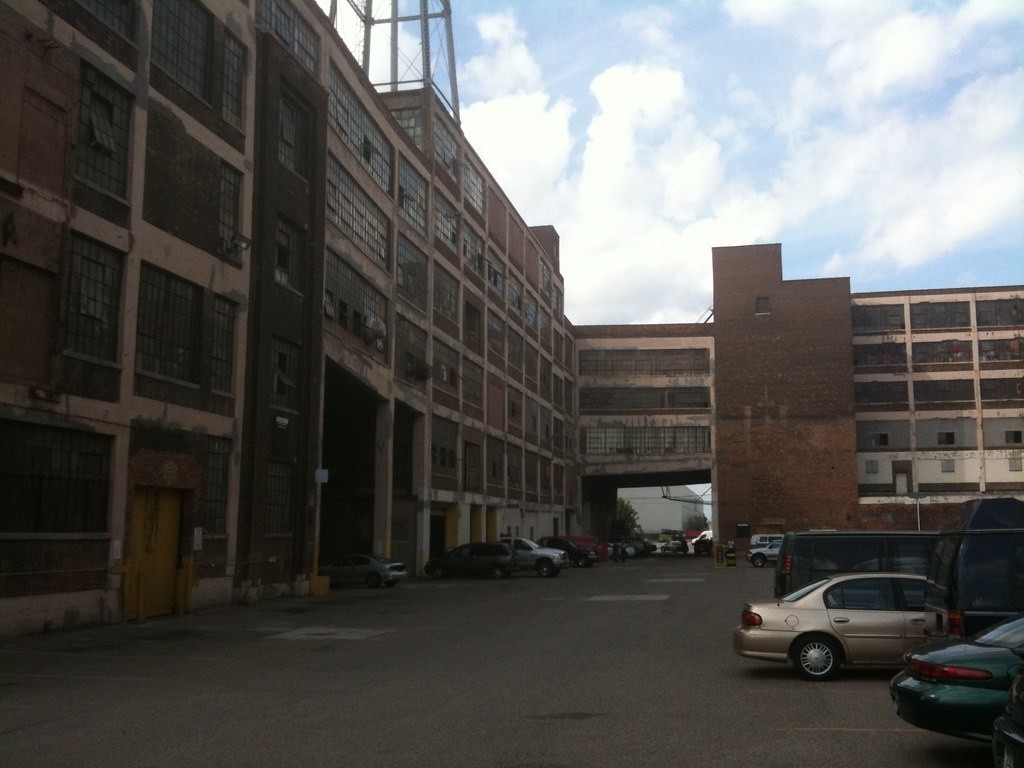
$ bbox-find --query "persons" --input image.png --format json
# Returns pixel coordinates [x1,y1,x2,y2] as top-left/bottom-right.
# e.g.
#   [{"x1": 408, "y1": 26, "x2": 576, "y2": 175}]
[
  {"x1": 612, "y1": 540, "x2": 627, "y2": 562},
  {"x1": 728, "y1": 537, "x2": 734, "y2": 553},
  {"x1": 681, "y1": 538, "x2": 688, "y2": 556}
]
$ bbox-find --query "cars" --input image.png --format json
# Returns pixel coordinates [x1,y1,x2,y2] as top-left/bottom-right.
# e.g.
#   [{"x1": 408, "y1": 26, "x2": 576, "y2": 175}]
[
  {"x1": 691, "y1": 531, "x2": 713, "y2": 557},
  {"x1": 732, "y1": 573, "x2": 940, "y2": 683},
  {"x1": 847, "y1": 555, "x2": 926, "y2": 574},
  {"x1": 661, "y1": 541, "x2": 689, "y2": 553},
  {"x1": 319, "y1": 553, "x2": 409, "y2": 588},
  {"x1": 576, "y1": 536, "x2": 658, "y2": 561},
  {"x1": 888, "y1": 612, "x2": 1024, "y2": 742}
]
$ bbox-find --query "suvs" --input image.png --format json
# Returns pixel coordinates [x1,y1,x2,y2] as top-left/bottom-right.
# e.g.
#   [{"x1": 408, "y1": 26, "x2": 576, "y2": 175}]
[
  {"x1": 423, "y1": 541, "x2": 521, "y2": 579},
  {"x1": 747, "y1": 540, "x2": 782, "y2": 568},
  {"x1": 537, "y1": 537, "x2": 598, "y2": 568},
  {"x1": 496, "y1": 537, "x2": 570, "y2": 578}
]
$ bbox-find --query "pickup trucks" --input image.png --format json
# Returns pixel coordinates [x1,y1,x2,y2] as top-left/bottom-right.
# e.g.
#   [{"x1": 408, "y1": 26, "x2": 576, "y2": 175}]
[{"x1": 774, "y1": 529, "x2": 942, "y2": 594}]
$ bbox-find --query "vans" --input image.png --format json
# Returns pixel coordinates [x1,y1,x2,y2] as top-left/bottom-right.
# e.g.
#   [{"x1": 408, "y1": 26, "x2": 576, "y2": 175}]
[
  {"x1": 921, "y1": 498, "x2": 1023, "y2": 643},
  {"x1": 750, "y1": 534, "x2": 785, "y2": 549}
]
